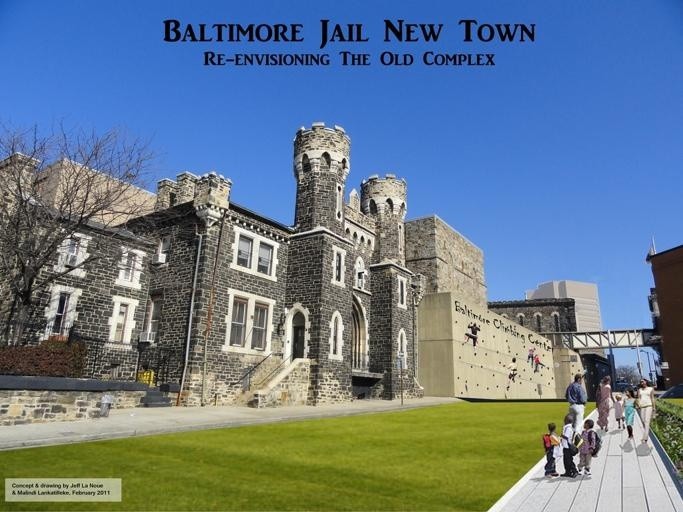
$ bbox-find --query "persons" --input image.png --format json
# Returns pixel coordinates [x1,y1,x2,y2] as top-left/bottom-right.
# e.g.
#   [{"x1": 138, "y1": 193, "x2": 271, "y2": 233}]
[
  {"x1": 620, "y1": 389, "x2": 639, "y2": 437},
  {"x1": 533, "y1": 354, "x2": 545, "y2": 373},
  {"x1": 507, "y1": 357, "x2": 518, "y2": 388},
  {"x1": 577, "y1": 419, "x2": 597, "y2": 475},
  {"x1": 595, "y1": 375, "x2": 616, "y2": 431},
  {"x1": 559, "y1": 414, "x2": 578, "y2": 478},
  {"x1": 543, "y1": 423, "x2": 561, "y2": 478},
  {"x1": 463, "y1": 322, "x2": 480, "y2": 353},
  {"x1": 526, "y1": 347, "x2": 536, "y2": 369},
  {"x1": 614, "y1": 394, "x2": 626, "y2": 430},
  {"x1": 637, "y1": 379, "x2": 656, "y2": 441}
]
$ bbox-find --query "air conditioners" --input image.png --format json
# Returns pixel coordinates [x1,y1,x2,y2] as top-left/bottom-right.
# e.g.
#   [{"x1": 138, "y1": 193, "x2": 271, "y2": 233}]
[
  {"x1": 65, "y1": 254, "x2": 79, "y2": 268},
  {"x1": 151, "y1": 253, "x2": 166, "y2": 264},
  {"x1": 139, "y1": 331, "x2": 157, "y2": 342}
]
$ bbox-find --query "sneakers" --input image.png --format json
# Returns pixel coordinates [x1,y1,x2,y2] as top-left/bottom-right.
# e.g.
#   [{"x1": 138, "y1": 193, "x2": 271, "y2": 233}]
[{"x1": 545, "y1": 469, "x2": 590, "y2": 479}]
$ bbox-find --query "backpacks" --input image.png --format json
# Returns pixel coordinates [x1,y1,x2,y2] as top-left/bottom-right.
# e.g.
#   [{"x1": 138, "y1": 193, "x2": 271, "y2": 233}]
[
  {"x1": 543, "y1": 433, "x2": 552, "y2": 454},
  {"x1": 561, "y1": 430, "x2": 583, "y2": 456},
  {"x1": 588, "y1": 430, "x2": 602, "y2": 457}
]
